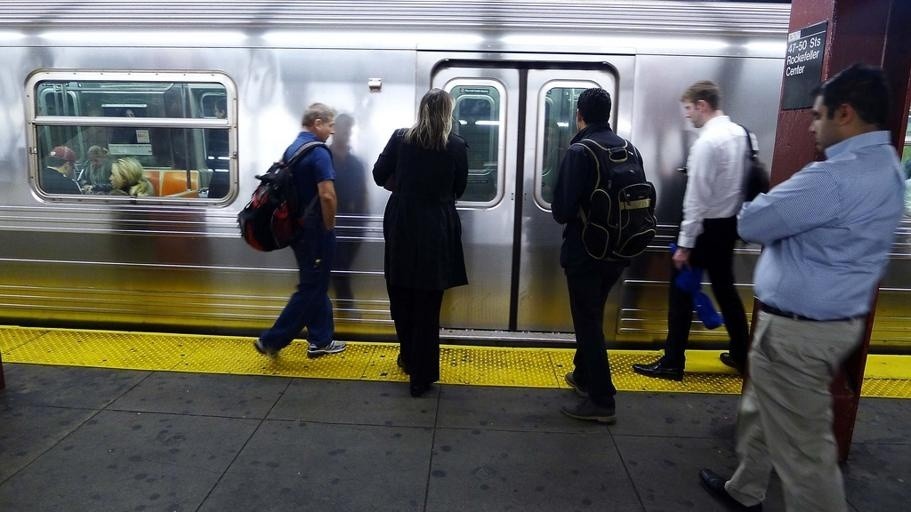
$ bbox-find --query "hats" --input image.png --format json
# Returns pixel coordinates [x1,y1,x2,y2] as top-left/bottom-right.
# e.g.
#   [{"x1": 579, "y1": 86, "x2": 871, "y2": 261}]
[{"x1": 48, "y1": 146, "x2": 79, "y2": 163}]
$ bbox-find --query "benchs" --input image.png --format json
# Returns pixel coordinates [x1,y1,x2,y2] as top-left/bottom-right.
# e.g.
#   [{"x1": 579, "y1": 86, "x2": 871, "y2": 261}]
[{"x1": 144, "y1": 169, "x2": 199, "y2": 198}]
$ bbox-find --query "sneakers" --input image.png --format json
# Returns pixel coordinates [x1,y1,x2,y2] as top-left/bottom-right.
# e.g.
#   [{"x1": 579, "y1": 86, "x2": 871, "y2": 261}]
[
  {"x1": 566, "y1": 370, "x2": 591, "y2": 397},
  {"x1": 253, "y1": 337, "x2": 281, "y2": 358},
  {"x1": 557, "y1": 395, "x2": 618, "y2": 424},
  {"x1": 306, "y1": 338, "x2": 347, "y2": 358},
  {"x1": 406, "y1": 372, "x2": 433, "y2": 399}
]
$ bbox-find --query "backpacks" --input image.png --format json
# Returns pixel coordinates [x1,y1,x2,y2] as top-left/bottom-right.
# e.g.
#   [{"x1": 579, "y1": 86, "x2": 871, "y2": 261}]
[
  {"x1": 235, "y1": 140, "x2": 333, "y2": 253},
  {"x1": 578, "y1": 138, "x2": 658, "y2": 265}
]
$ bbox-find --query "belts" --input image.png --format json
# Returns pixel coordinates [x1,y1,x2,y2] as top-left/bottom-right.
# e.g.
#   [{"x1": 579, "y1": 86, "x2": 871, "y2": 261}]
[{"x1": 755, "y1": 299, "x2": 871, "y2": 325}]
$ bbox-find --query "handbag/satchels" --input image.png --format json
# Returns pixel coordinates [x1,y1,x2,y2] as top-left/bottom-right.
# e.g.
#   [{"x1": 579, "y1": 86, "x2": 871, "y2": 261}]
[{"x1": 739, "y1": 123, "x2": 770, "y2": 204}]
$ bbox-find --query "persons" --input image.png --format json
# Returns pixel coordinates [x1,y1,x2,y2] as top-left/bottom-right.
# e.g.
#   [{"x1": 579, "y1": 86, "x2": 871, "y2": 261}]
[
  {"x1": 696, "y1": 57, "x2": 907, "y2": 512},
  {"x1": 42, "y1": 146, "x2": 154, "y2": 197},
  {"x1": 328, "y1": 112, "x2": 368, "y2": 322},
  {"x1": 252, "y1": 102, "x2": 348, "y2": 358},
  {"x1": 632, "y1": 80, "x2": 759, "y2": 381},
  {"x1": 372, "y1": 88, "x2": 471, "y2": 398},
  {"x1": 552, "y1": 88, "x2": 646, "y2": 423}
]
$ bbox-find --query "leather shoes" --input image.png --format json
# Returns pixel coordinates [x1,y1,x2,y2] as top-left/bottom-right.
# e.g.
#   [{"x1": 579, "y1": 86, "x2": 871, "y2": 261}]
[
  {"x1": 720, "y1": 354, "x2": 732, "y2": 367},
  {"x1": 699, "y1": 467, "x2": 763, "y2": 512},
  {"x1": 632, "y1": 359, "x2": 683, "y2": 381}
]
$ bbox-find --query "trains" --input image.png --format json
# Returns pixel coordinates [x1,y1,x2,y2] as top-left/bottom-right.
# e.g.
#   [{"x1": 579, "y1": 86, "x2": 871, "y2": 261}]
[{"x1": 1, "y1": 0, "x2": 911, "y2": 356}]
